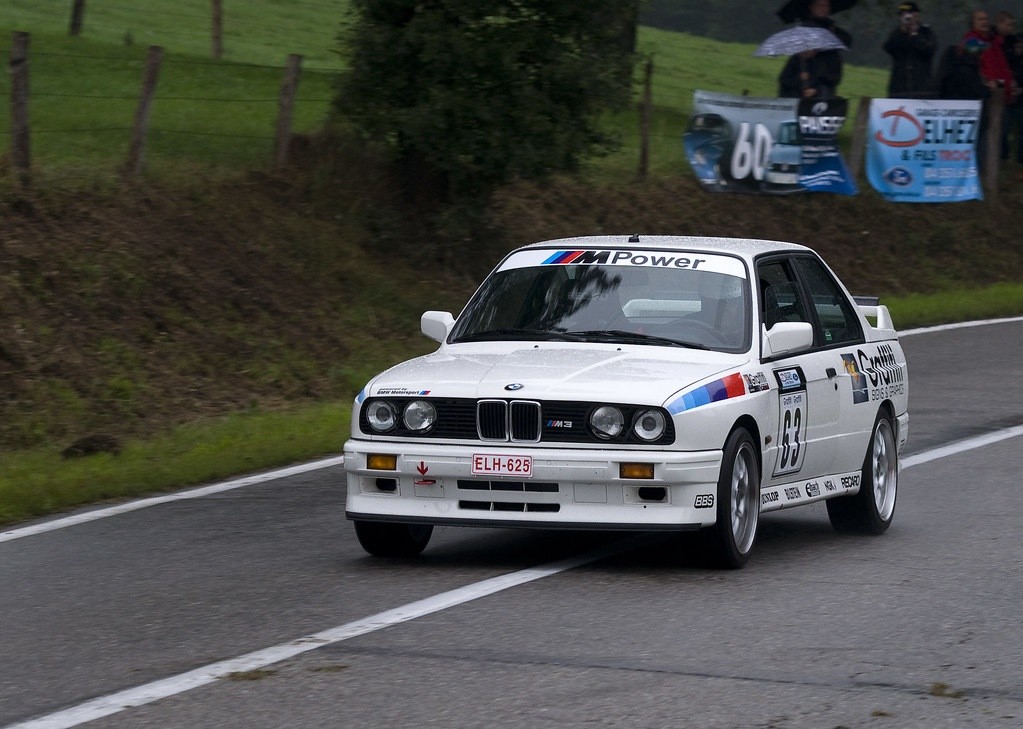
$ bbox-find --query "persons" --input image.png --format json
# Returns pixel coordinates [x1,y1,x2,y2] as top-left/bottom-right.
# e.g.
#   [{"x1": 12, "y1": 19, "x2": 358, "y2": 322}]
[
  {"x1": 776, "y1": 42, "x2": 819, "y2": 99},
  {"x1": 999, "y1": 32, "x2": 1023, "y2": 165},
  {"x1": 881, "y1": 2, "x2": 942, "y2": 95},
  {"x1": 661, "y1": 271, "x2": 743, "y2": 350},
  {"x1": 793, "y1": 0, "x2": 851, "y2": 75},
  {"x1": 990, "y1": 13, "x2": 1014, "y2": 61},
  {"x1": 937, "y1": 11, "x2": 1009, "y2": 161}
]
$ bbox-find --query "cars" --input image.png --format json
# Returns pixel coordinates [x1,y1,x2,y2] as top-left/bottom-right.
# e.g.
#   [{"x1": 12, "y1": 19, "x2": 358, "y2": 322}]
[{"x1": 337, "y1": 231, "x2": 912, "y2": 569}]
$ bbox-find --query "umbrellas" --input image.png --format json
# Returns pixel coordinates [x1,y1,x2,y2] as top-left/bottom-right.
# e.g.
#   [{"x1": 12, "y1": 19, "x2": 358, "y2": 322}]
[
  {"x1": 753, "y1": 26, "x2": 848, "y2": 57},
  {"x1": 773, "y1": 1, "x2": 858, "y2": 22}
]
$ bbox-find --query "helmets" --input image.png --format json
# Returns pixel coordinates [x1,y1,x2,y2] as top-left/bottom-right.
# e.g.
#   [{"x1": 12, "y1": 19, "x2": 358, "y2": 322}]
[{"x1": 699, "y1": 272, "x2": 741, "y2": 299}]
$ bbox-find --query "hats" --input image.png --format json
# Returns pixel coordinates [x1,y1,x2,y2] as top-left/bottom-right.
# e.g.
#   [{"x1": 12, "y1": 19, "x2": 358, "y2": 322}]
[{"x1": 894, "y1": 2, "x2": 920, "y2": 14}]
